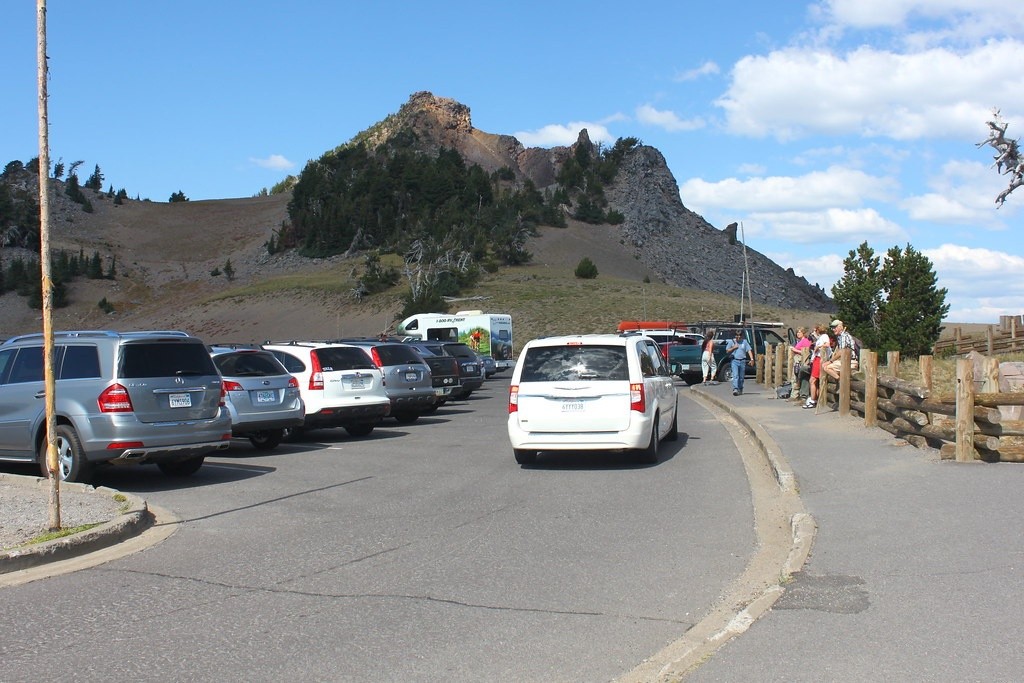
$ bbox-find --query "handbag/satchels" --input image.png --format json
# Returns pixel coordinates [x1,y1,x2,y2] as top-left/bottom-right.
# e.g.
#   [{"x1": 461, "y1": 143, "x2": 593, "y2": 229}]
[
  {"x1": 728, "y1": 353, "x2": 734, "y2": 359},
  {"x1": 794, "y1": 365, "x2": 799, "y2": 374},
  {"x1": 800, "y1": 355, "x2": 811, "y2": 374}
]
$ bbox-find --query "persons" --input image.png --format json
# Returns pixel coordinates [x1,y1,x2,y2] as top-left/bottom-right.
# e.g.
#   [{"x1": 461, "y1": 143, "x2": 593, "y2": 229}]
[
  {"x1": 785, "y1": 327, "x2": 811, "y2": 402},
  {"x1": 802, "y1": 323, "x2": 830, "y2": 409},
  {"x1": 726, "y1": 329, "x2": 755, "y2": 396},
  {"x1": 470, "y1": 328, "x2": 482, "y2": 349},
  {"x1": 821, "y1": 319, "x2": 858, "y2": 394},
  {"x1": 701, "y1": 331, "x2": 717, "y2": 385}
]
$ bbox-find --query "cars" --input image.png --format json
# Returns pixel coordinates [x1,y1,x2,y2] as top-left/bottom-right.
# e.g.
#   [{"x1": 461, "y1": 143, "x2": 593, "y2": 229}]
[{"x1": 470, "y1": 348, "x2": 497, "y2": 379}]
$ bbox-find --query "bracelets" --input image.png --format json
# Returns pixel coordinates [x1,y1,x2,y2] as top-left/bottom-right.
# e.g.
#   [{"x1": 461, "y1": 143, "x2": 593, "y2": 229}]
[{"x1": 830, "y1": 361, "x2": 833, "y2": 363}]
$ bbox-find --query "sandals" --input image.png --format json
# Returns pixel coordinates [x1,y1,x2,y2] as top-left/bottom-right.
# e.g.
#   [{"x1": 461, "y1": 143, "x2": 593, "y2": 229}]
[{"x1": 802, "y1": 401, "x2": 817, "y2": 409}]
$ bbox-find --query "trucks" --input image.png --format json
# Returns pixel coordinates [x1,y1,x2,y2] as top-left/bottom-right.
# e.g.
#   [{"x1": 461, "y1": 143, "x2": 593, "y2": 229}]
[{"x1": 396, "y1": 309, "x2": 517, "y2": 372}]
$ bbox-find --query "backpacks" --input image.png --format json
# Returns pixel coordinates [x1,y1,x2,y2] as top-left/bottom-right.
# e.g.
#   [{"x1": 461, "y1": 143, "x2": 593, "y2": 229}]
[{"x1": 844, "y1": 330, "x2": 863, "y2": 361}]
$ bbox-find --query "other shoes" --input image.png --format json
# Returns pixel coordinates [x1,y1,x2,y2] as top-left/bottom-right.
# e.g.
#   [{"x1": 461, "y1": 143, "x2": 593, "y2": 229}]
[
  {"x1": 703, "y1": 382, "x2": 707, "y2": 386},
  {"x1": 733, "y1": 389, "x2": 739, "y2": 395},
  {"x1": 709, "y1": 381, "x2": 715, "y2": 386},
  {"x1": 786, "y1": 394, "x2": 799, "y2": 402},
  {"x1": 739, "y1": 388, "x2": 743, "y2": 395}
]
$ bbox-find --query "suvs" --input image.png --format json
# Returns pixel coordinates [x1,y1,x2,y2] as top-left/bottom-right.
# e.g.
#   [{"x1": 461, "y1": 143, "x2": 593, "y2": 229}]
[
  {"x1": 402, "y1": 340, "x2": 464, "y2": 414},
  {"x1": 420, "y1": 341, "x2": 486, "y2": 400},
  {"x1": 326, "y1": 336, "x2": 437, "y2": 422},
  {"x1": 205, "y1": 343, "x2": 306, "y2": 452},
  {"x1": 505, "y1": 333, "x2": 682, "y2": 465},
  {"x1": 0, "y1": 330, "x2": 233, "y2": 482},
  {"x1": 219, "y1": 340, "x2": 392, "y2": 436}
]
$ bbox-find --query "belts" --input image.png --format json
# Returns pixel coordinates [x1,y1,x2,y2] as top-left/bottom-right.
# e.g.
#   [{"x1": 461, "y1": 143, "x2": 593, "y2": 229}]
[{"x1": 734, "y1": 359, "x2": 746, "y2": 361}]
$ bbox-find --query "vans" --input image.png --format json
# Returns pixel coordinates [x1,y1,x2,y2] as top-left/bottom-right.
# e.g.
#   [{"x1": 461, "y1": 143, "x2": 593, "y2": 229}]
[{"x1": 622, "y1": 328, "x2": 706, "y2": 345}]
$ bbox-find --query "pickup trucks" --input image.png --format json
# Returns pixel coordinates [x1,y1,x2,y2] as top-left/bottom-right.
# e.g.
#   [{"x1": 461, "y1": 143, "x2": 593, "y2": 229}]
[{"x1": 661, "y1": 326, "x2": 787, "y2": 384}]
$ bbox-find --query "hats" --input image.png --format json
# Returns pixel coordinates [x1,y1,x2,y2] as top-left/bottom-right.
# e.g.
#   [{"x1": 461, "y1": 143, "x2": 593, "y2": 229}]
[{"x1": 829, "y1": 319, "x2": 844, "y2": 327}]
